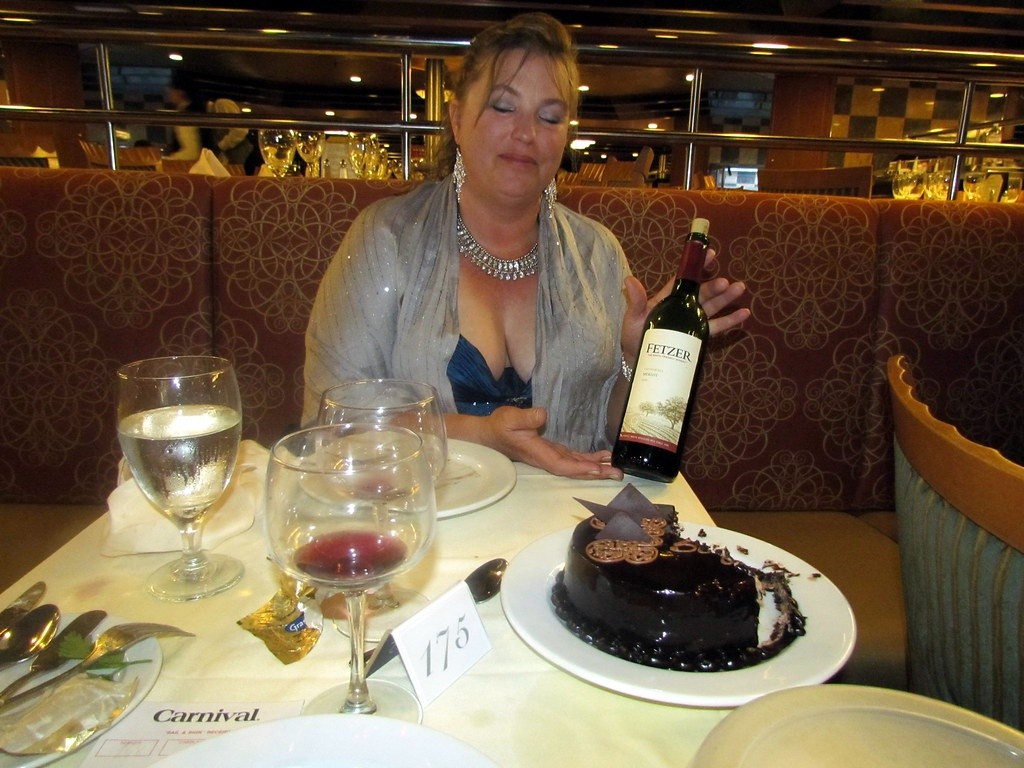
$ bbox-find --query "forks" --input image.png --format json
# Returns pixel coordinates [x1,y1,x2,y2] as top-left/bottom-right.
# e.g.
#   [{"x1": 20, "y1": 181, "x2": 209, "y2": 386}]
[{"x1": 0, "y1": 623, "x2": 198, "y2": 725}]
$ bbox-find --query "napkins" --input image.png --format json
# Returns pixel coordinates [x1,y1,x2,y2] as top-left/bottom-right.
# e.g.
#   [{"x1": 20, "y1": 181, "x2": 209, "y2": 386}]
[{"x1": 100, "y1": 461, "x2": 259, "y2": 558}]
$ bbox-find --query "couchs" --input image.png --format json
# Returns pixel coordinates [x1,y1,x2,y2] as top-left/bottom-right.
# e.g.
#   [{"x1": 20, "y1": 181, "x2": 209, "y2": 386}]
[{"x1": 2, "y1": 159, "x2": 1022, "y2": 694}]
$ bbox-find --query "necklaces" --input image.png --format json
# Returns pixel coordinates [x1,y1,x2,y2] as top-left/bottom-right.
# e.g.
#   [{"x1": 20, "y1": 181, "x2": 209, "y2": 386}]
[{"x1": 457, "y1": 208, "x2": 538, "y2": 279}]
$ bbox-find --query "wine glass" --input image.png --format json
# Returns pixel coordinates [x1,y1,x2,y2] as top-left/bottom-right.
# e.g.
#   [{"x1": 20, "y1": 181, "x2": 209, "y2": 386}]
[
  {"x1": 891, "y1": 164, "x2": 1022, "y2": 203},
  {"x1": 119, "y1": 354, "x2": 246, "y2": 601},
  {"x1": 258, "y1": 129, "x2": 425, "y2": 179},
  {"x1": 264, "y1": 382, "x2": 448, "y2": 720}
]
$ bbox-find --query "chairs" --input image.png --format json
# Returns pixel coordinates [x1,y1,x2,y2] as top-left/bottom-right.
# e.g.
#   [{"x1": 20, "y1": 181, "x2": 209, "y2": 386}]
[{"x1": 885, "y1": 352, "x2": 1024, "y2": 729}]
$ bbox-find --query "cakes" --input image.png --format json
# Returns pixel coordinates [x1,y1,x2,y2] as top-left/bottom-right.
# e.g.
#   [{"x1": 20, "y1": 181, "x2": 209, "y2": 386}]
[{"x1": 548, "y1": 497, "x2": 807, "y2": 673}]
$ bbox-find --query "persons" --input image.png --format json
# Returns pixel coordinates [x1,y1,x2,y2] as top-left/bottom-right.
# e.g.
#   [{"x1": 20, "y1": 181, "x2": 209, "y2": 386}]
[
  {"x1": 300, "y1": 14, "x2": 752, "y2": 483},
  {"x1": 156, "y1": 78, "x2": 219, "y2": 175},
  {"x1": 207, "y1": 99, "x2": 254, "y2": 174}
]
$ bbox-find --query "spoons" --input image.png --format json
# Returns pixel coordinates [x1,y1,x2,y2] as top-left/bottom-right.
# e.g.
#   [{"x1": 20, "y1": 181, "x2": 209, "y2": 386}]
[{"x1": 0, "y1": 603, "x2": 60, "y2": 675}]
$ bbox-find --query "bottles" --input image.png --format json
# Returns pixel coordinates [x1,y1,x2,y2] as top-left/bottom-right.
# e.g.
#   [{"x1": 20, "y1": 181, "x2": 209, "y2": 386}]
[{"x1": 610, "y1": 219, "x2": 713, "y2": 483}]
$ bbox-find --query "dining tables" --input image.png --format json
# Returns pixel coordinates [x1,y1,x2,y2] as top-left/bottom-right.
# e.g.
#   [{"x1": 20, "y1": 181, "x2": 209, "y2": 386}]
[{"x1": 0, "y1": 450, "x2": 740, "y2": 768}]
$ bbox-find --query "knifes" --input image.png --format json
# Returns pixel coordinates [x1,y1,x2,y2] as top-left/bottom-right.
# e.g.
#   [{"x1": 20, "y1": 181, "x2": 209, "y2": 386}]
[
  {"x1": 0, "y1": 581, "x2": 46, "y2": 633},
  {"x1": 0, "y1": 609, "x2": 108, "y2": 706}
]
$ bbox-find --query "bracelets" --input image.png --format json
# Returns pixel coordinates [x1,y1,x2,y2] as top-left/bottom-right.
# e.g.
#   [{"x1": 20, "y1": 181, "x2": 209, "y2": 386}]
[{"x1": 621, "y1": 352, "x2": 637, "y2": 383}]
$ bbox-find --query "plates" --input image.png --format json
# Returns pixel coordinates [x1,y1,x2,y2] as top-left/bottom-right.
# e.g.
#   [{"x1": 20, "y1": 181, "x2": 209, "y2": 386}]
[
  {"x1": 298, "y1": 432, "x2": 516, "y2": 519},
  {"x1": 500, "y1": 521, "x2": 857, "y2": 708},
  {"x1": 0, "y1": 614, "x2": 164, "y2": 768},
  {"x1": 146, "y1": 713, "x2": 501, "y2": 768}
]
